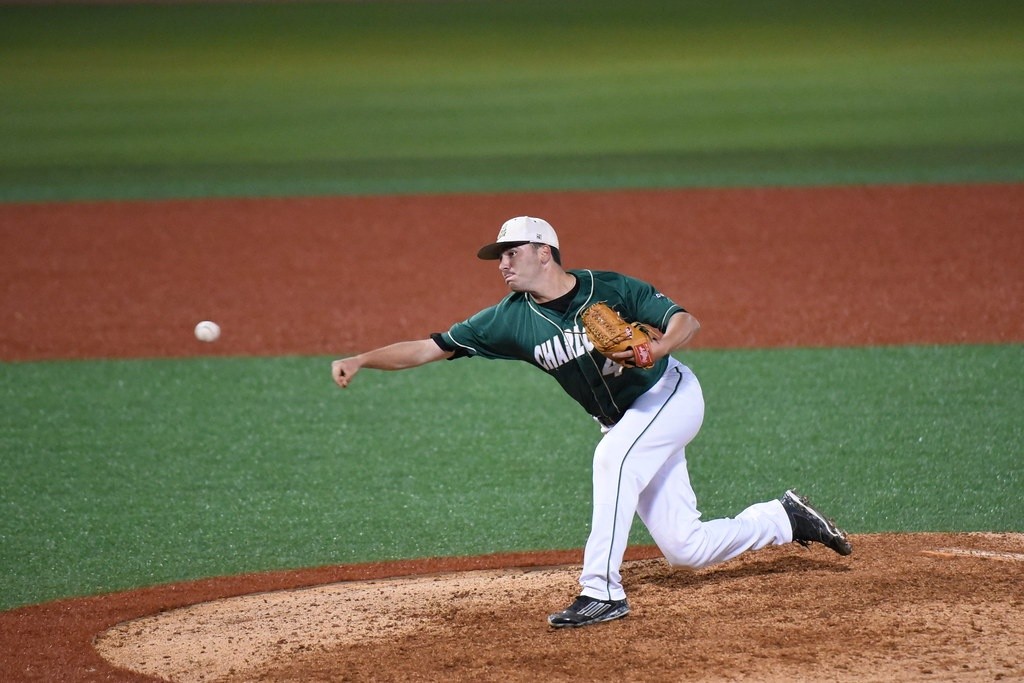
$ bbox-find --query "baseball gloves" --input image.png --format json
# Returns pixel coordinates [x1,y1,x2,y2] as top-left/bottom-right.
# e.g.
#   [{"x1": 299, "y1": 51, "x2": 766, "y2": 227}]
[{"x1": 580, "y1": 305, "x2": 661, "y2": 369}]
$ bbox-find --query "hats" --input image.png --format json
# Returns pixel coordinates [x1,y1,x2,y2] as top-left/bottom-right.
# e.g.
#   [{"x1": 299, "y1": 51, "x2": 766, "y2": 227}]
[{"x1": 477, "y1": 216, "x2": 560, "y2": 260}]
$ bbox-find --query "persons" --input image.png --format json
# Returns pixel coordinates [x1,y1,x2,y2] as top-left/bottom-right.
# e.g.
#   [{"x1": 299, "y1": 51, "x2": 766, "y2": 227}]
[{"x1": 332, "y1": 217, "x2": 853, "y2": 627}]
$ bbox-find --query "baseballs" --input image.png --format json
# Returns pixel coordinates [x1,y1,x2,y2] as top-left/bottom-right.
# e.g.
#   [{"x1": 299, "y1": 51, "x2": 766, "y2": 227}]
[{"x1": 194, "y1": 322, "x2": 220, "y2": 342}]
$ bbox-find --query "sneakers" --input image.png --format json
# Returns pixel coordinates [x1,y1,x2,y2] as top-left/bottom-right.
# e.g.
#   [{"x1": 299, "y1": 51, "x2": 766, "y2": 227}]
[
  {"x1": 548, "y1": 595, "x2": 630, "y2": 627},
  {"x1": 780, "y1": 490, "x2": 852, "y2": 556}
]
